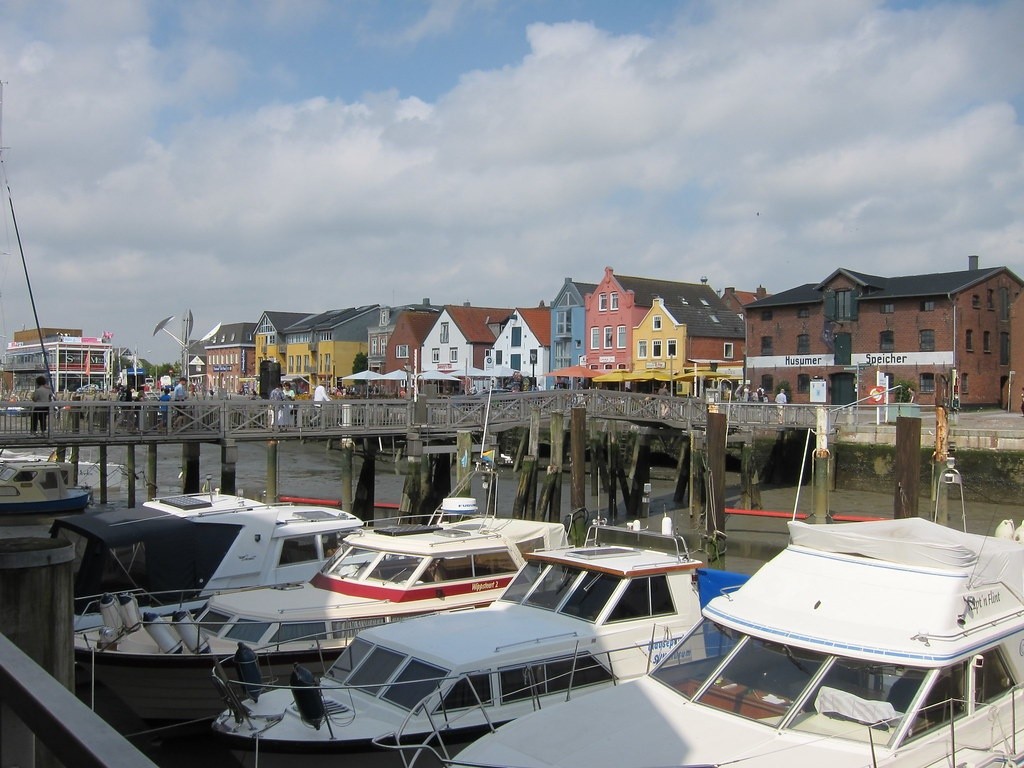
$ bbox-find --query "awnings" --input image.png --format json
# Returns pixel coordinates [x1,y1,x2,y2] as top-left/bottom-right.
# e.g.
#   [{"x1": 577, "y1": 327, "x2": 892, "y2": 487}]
[{"x1": 281, "y1": 373, "x2": 307, "y2": 381}]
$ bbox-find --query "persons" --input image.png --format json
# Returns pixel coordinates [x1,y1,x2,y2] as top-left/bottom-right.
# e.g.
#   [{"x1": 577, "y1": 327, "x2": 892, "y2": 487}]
[
  {"x1": 268, "y1": 381, "x2": 294, "y2": 432},
  {"x1": 626, "y1": 387, "x2": 632, "y2": 392},
  {"x1": 775, "y1": 389, "x2": 787, "y2": 424},
  {"x1": 332, "y1": 384, "x2": 385, "y2": 396},
  {"x1": 30, "y1": 376, "x2": 52, "y2": 436},
  {"x1": 657, "y1": 382, "x2": 671, "y2": 419},
  {"x1": 110, "y1": 378, "x2": 188, "y2": 431},
  {"x1": 309, "y1": 381, "x2": 330, "y2": 425},
  {"x1": 908, "y1": 387, "x2": 916, "y2": 403},
  {"x1": 744, "y1": 385, "x2": 768, "y2": 403}
]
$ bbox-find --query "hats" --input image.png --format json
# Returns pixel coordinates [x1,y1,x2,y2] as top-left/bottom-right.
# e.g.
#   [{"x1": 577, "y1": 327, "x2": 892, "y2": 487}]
[
  {"x1": 179, "y1": 376, "x2": 189, "y2": 382},
  {"x1": 319, "y1": 380, "x2": 326, "y2": 385},
  {"x1": 282, "y1": 381, "x2": 290, "y2": 386}
]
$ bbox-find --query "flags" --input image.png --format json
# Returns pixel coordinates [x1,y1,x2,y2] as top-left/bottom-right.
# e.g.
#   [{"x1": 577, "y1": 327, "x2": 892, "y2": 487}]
[
  {"x1": 103, "y1": 331, "x2": 112, "y2": 339},
  {"x1": 84, "y1": 351, "x2": 90, "y2": 376},
  {"x1": 480, "y1": 450, "x2": 494, "y2": 462}
]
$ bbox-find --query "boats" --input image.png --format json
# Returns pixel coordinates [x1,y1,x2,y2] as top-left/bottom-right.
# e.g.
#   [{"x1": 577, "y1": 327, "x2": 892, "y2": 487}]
[
  {"x1": 73, "y1": 382, "x2": 573, "y2": 737},
  {"x1": 366, "y1": 427, "x2": 1023, "y2": 768},
  {"x1": 212, "y1": 468, "x2": 749, "y2": 768},
  {"x1": 0, "y1": 406, "x2": 31, "y2": 416},
  {"x1": 49, "y1": 489, "x2": 366, "y2": 615},
  {"x1": 0, "y1": 449, "x2": 123, "y2": 516}
]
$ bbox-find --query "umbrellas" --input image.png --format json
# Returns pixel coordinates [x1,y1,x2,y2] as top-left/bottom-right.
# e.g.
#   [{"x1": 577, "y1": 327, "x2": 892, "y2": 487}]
[
  {"x1": 340, "y1": 365, "x2": 520, "y2": 398},
  {"x1": 541, "y1": 365, "x2": 731, "y2": 392}
]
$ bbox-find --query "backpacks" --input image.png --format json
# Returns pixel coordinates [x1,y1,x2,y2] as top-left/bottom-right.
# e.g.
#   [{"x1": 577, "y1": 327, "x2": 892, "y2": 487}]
[{"x1": 757, "y1": 388, "x2": 763, "y2": 396}]
